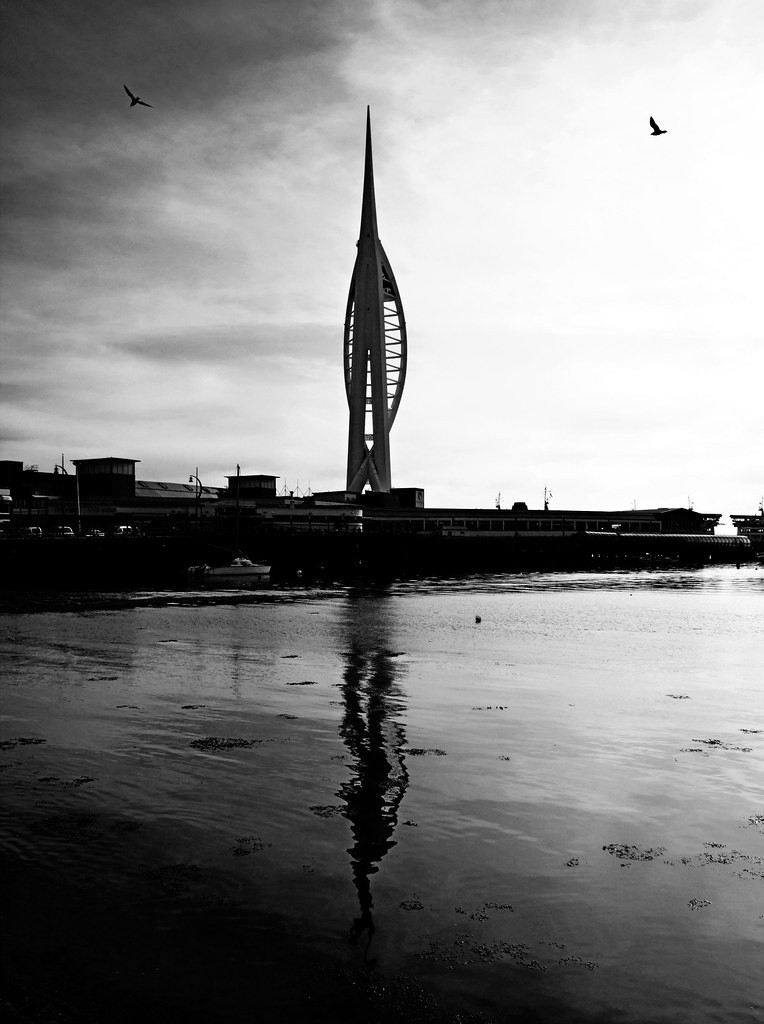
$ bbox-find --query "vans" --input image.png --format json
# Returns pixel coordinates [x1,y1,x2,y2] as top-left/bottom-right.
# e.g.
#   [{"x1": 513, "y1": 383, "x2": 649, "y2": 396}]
[{"x1": 110, "y1": 525, "x2": 135, "y2": 536}]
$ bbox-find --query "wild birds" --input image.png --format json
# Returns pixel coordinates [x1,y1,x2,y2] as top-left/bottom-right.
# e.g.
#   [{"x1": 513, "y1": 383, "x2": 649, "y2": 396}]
[
  {"x1": 649, "y1": 115, "x2": 667, "y2": 138},
  {"x1": 124, "y1": 84, "x2": 154, "y2": 109}
]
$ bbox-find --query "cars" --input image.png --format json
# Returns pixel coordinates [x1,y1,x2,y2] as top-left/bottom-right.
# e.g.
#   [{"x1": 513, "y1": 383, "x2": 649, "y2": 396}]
[
  {"x1": 83, "y1": 529, "x2": 107, "y2": 537},
  {"x1": 15, "y1": 527, "x2": 42, "y2": 539},
  {"x1": 56, "y1": 525, "x2": 76, "y2": 537}
]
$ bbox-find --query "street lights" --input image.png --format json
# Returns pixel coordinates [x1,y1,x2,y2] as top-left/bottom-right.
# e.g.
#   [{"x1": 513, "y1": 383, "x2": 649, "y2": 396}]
[
  {"x1": 74, "y1": 461, "x2": 84, "y2": 536},
  {"x1": 188, "y1": 476, "x2": 204, "y2": 520},
  {"x1": 236, "y1": 463, "x2": 240, "y2": 513}
]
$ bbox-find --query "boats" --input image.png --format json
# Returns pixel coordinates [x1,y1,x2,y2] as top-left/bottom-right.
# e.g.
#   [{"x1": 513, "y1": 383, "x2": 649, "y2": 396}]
[{"x1": 188, "y1": 557, "x2": 273, "y2": 576}]
